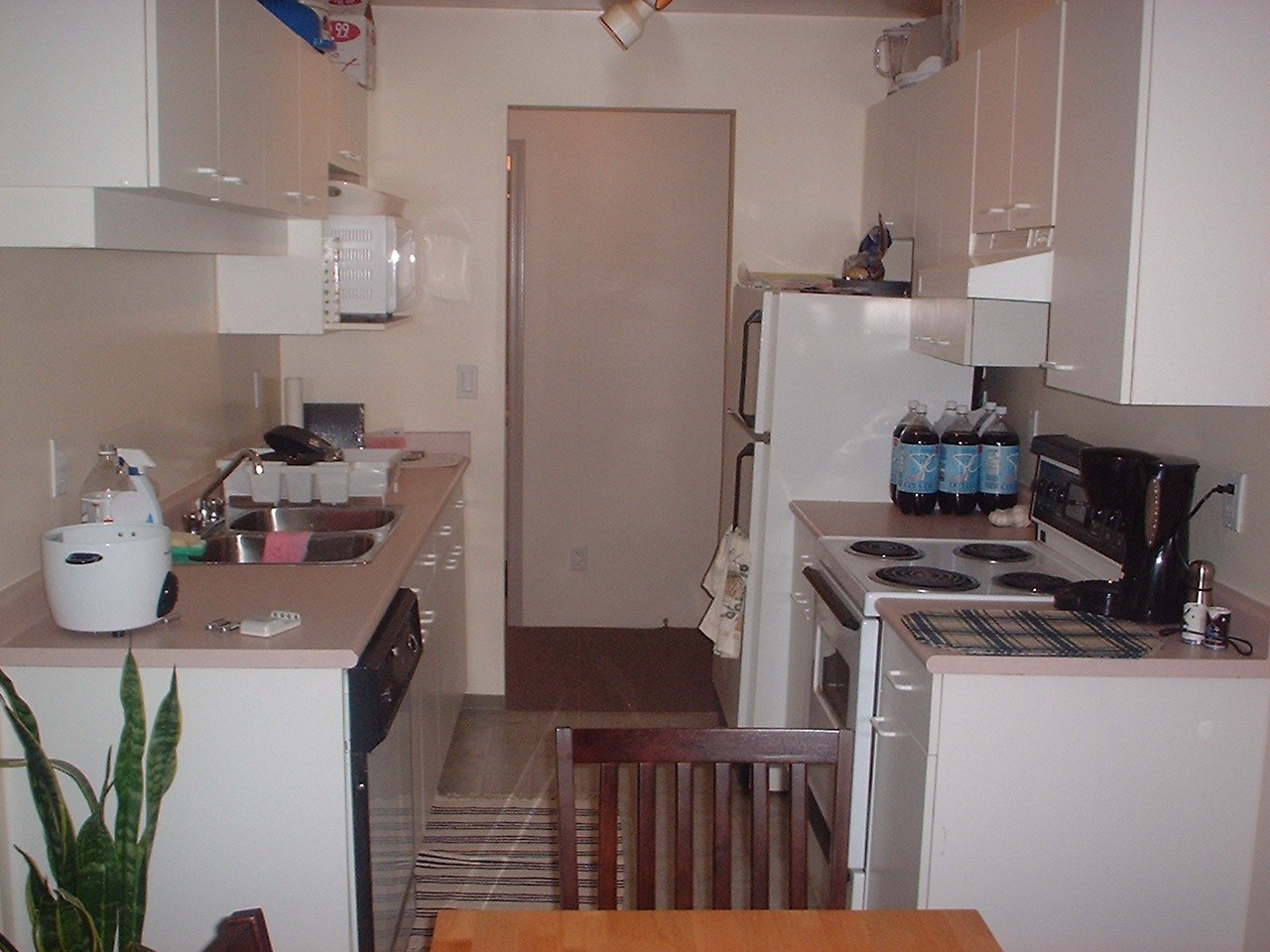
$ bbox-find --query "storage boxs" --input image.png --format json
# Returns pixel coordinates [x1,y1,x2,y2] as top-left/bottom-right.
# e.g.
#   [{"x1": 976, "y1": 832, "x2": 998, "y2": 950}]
[{"x1": 306, "y1": 0, "x2": 377, "y2": 92}]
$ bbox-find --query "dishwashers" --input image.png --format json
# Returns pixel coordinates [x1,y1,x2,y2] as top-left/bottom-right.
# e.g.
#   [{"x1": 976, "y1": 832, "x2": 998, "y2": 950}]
[{"x1": 343, "y1": 588, "x2": 423, "y2": 952}]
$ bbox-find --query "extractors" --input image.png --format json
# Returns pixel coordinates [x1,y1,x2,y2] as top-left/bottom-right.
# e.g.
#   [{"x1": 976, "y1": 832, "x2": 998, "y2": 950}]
[{"x1": 908, "y1": 225, "x2": 1054, "y2": 366}]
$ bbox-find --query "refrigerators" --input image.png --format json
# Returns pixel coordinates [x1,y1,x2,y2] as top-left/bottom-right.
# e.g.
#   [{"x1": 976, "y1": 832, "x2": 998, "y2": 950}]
[{"x1": 710, "y1": 281, "x2": 973, "y2": 791}]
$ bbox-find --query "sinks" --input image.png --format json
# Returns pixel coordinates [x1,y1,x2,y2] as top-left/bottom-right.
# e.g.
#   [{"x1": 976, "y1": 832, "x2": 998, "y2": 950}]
[
  {"x1": 224, "y1": 503, "x2": 402, "y2": 542},
  {"x1": 187, "y1": 528, "x2": 384, "y2": 567}
]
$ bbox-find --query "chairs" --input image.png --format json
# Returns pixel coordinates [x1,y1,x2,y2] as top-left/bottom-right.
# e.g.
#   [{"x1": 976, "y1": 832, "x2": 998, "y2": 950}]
[{"x1": 555, "y1": 725, "x2": 856, "y2": 911}]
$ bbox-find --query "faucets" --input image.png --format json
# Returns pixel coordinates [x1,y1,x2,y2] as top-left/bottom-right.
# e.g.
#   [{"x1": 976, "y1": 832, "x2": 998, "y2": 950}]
[{"x1": 182, "y1": 448, "x2": 264, "y2": 539}]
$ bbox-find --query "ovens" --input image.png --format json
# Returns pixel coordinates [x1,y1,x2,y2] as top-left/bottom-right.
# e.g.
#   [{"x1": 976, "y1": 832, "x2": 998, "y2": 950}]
[{"x1": 799, "y1": 557, "x2": 879, "y2": 913}]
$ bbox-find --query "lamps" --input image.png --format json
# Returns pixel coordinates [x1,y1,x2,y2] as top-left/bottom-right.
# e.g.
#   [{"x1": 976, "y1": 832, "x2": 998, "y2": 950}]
[{"x1": 598, "y1": 0, "x2": 671, "y2": 51}]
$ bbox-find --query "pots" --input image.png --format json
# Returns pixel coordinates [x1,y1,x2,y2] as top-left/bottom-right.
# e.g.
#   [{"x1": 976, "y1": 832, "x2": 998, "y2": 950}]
[{"x1": 263, "y1": 425, "x2": 343, "y2": 465}]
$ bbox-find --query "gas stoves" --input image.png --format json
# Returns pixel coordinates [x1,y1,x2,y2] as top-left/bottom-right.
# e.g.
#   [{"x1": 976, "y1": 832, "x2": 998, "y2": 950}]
[{"x1": 814, "y1": 434, "x2": 1093, "y2": 617}]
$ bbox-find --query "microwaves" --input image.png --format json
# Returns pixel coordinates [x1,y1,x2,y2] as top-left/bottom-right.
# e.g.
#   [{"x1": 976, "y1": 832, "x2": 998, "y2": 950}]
[{"x1": 332, "y1": 216, "x2": 418, "y2": 323}]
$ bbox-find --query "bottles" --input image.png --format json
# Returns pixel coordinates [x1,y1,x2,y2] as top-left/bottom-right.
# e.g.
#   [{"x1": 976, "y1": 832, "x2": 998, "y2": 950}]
[
  {"x1": 1187, "y1": 558, "x2": 1214, "y2": 607},
  {"x1": 77, "y1": 443, "x2": 139, "y2": 523},
  {"x1": 887, "y1": 399, "x2": 1019, "y2": 517}
]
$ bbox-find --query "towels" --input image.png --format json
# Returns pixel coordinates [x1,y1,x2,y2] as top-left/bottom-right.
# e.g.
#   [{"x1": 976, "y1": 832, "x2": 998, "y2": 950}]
[{"x1": 698, "y1": 525, "x2": 750, "y2": 661}]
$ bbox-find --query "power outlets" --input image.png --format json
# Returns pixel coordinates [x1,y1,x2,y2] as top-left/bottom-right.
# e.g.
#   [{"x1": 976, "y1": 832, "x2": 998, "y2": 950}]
[{"x1": 1220, "y1": 469, "x2": 1246, "y2": 533}]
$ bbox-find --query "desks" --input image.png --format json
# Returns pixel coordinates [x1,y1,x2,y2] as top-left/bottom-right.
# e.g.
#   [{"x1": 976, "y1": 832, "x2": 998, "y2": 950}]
[{"x1": 430, "y1": 908, "x2": 1004, "y2": 952}]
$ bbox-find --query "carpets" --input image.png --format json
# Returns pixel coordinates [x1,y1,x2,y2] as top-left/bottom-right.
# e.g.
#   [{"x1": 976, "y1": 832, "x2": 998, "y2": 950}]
[
  {"x1": 401, "y1": 791, "x2": 625, "y2": 952},
  {"x1": 504, "y1": 626, "x2": 718, "y2": 710}
]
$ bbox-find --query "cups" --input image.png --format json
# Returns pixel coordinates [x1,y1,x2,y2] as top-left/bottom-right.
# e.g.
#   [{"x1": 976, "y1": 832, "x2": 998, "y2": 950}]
[
  {"x1": 875, "y1": 28, "x2": 911, "y2": 77},
  {"x1": 1204, "y1": 608, "x2": 1232, "y2": 651},
  {"x1": 1180, "y1": 602, "x2": 1206, "y2": 645}
]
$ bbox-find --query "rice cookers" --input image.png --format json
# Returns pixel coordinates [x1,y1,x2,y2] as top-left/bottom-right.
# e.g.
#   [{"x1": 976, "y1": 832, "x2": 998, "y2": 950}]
[{"x1": 41, "y1": 521, "x2": 179, "y2": 637}]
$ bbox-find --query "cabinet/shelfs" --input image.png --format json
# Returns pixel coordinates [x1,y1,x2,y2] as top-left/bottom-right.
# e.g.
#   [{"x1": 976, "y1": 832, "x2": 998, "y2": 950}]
[
  {"x1": 970, "y1": 1, "x2": 1065, "y2": 231},
  {"x1": 863, "y1": 618, "x2": 1269, "y2": 952},
  {"x1": 1038, "y1": 1, "x2": 1270, "y2": 406},
  {"x1": 263, "y1": 8, "x2": 329, "y2": 220},
  {"x1": 858, "y1": 84, "x2": 920, "y2": 237},
  {"x1": 398, "y1": 478, "x2": 468, "y2": 835},
  {"x1": 910, "y1": 49, "x2": 1049, "y2": 366},
  {"x1": 782, "y1": 516, "x2": 816, "y2": 730},
  {"x1": 329, "y1": 61, "x2": 369, "y2": 186},
  {"x1": 0, "y1": 0, "x2": 268, "y2": 253}
]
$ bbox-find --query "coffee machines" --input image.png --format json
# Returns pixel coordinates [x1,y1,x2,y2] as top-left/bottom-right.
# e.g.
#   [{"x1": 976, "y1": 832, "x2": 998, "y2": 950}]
[{"x1": 1056, "y1": 446, "x2": 1200, "y2": 623}]
